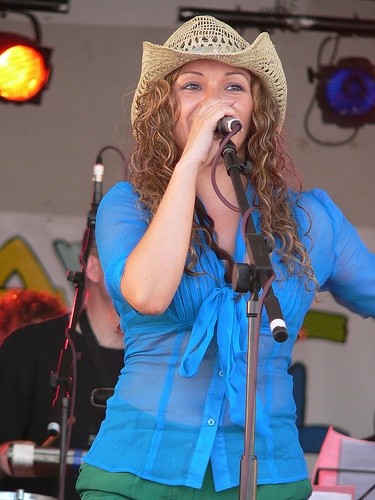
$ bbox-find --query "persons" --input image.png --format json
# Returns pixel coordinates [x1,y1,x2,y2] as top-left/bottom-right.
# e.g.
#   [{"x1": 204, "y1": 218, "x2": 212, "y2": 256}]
[
  {"x1": 1, "y1": 223, "x2": 126, "y2": 500},
  {"x1": 75, "y1": 16, "x2": 375, "y2": 500}
]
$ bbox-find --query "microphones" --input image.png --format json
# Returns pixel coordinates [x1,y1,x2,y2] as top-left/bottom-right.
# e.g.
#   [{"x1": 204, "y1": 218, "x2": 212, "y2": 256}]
[
  {"x1": 215, "y1": 116, "x2": 243, "y2": 133},
  {"x1": 87, "y1": 155, "x2": 105, "y2": 229}
]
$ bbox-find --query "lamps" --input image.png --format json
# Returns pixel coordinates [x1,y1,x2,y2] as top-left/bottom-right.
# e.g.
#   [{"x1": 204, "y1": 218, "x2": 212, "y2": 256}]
[
  {"x1": 0, "y1": 9, "x2": 54, "y2": 107},
  {"x1": 304, "y1": 34, "x2": 375, "y2": 147}
]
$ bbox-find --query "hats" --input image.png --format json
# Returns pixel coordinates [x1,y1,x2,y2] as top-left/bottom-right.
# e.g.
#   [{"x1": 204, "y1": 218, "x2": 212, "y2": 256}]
[{"x1": 131, "y1": 15, "x2": 288, "y2": 144}]
